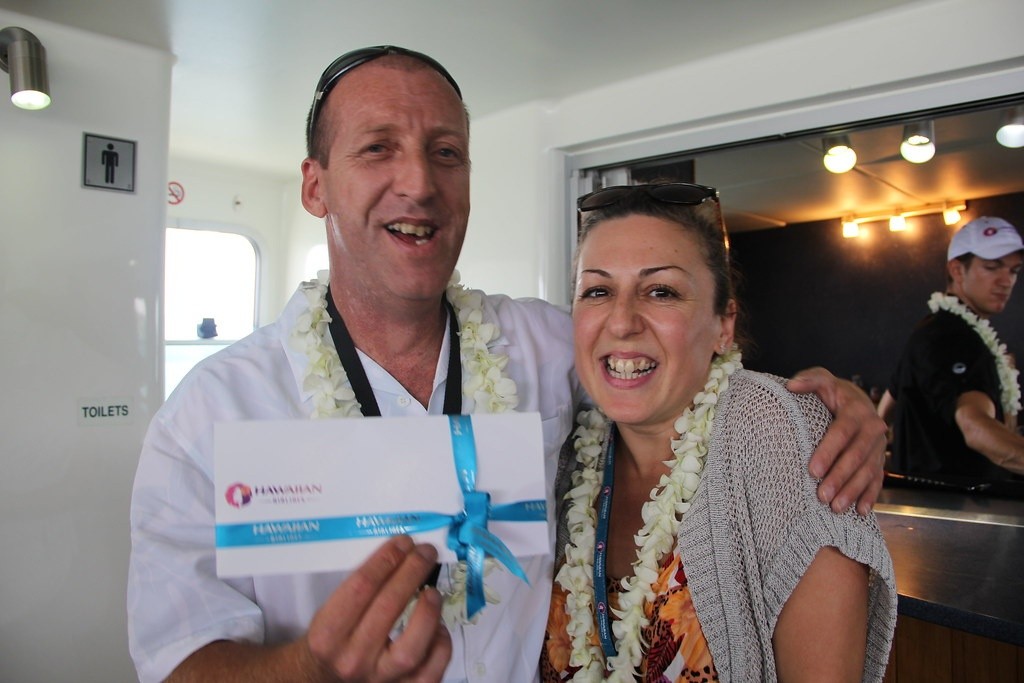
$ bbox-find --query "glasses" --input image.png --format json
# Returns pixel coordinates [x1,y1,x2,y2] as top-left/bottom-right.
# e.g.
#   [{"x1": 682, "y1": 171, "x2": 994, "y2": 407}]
[
  {"x1": 576, "y1": 180, "x2": 730, "y2": 275},
  {"x1": 306, "y1": 45, "x2": 465, "y2": 154}
]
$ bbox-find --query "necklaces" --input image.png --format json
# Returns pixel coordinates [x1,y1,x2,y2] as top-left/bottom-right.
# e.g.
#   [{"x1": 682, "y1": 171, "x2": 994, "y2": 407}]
[
  {"x1": 929, "y1": 291, "x2": 1023, "y2": 432},
  {"x1": 291, "y1": 265, "x2": 519, "y2": 642},
  {"x1": 555, "y1": 340, "x2": 743, "y2": 683}
]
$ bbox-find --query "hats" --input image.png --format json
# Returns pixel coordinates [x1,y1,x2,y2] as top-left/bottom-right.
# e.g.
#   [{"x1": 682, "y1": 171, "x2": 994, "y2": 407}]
[{"x1": 949, "y1": 215, "x2": 1024, "y2": 260}]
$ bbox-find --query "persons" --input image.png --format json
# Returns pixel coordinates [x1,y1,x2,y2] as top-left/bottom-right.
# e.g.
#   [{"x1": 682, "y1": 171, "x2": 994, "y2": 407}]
[
  {"x1": 121, "y1": 42, "x2": 896, "y2": 683},
  {"x1": 547, "y1": 181, "x2": 900, "y2": 683},
  {"x1": 878, "y1": 215, "x2": 1024, "y2": 501}
]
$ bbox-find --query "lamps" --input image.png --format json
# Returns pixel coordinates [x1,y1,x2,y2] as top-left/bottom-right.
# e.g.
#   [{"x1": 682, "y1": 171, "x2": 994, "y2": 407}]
[
  {"x1": 995, "y1": 103, "x2": 1024, "y2": 149},
  {"x1": 821, "y1": 134, "x2": 857, "y2": 176},
  {"x1": 0, "y1": 27, "x2": 51, "y2": 111},
  {"x1": 839, "y1": 198, "x2": 967, "y2": 238},
  {"x1": 897, "y1": 120, "x2": 937, "y2": 165}
]
